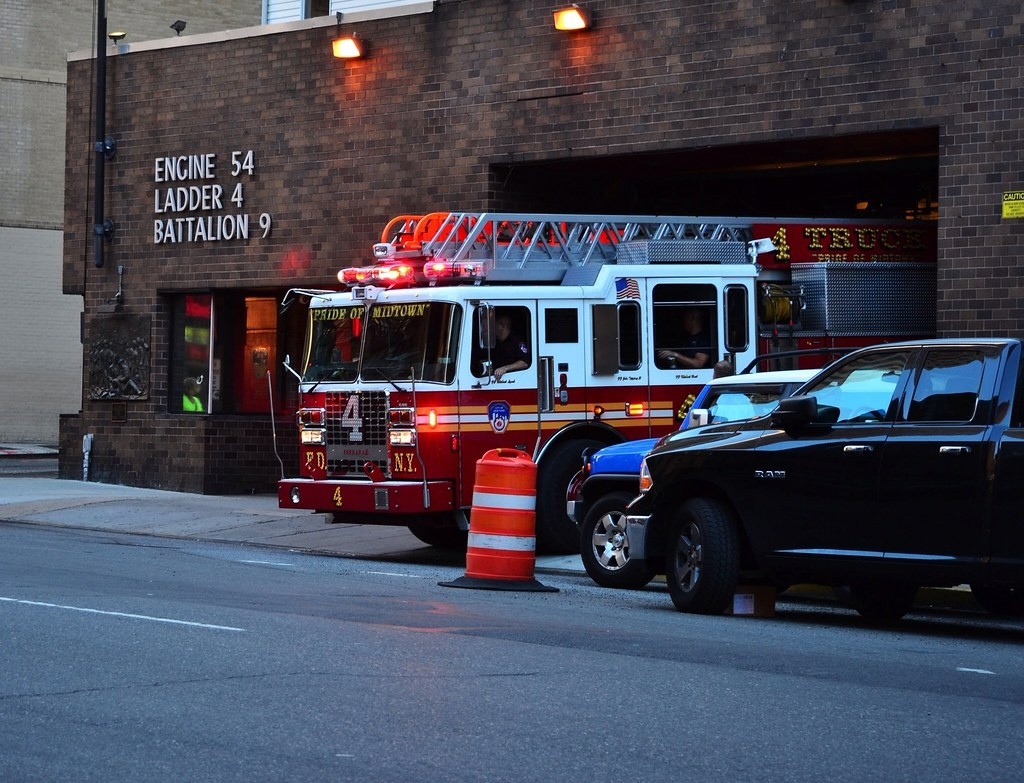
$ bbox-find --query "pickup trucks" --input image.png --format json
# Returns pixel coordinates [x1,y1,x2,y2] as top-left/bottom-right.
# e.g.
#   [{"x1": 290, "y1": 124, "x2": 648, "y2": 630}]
[{"x1": 626, "y1": 339, "x2": 1024, "y2": 629}]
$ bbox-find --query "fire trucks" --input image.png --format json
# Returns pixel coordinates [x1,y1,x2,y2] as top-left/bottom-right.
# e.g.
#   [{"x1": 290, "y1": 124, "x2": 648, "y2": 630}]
[{"x1": 276, "y1": 212, "x2": 937, "y2": 551}]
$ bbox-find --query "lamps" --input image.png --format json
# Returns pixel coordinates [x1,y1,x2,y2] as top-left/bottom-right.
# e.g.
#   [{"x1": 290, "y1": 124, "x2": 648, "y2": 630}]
[
  {"x1": 552, "y1": 7, "x2": 591, "y2": 30},
  {"x1": 331, "y1": 34, "x2": 367, "y2": 58},
  {"x1": 169, "y1": 20, "x2": 188, "y2": 35},
  {"x1": 108, "y1": 31, "x2": 125, "y2": 42}
]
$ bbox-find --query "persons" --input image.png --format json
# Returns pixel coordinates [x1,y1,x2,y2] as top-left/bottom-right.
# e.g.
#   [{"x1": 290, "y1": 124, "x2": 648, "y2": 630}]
[
  {"x1": 713, "y1": 360, "x2": 734, "y2": 379},
  {"x1": 476, "y1": 311, "x2": 531, "y2": 382},
  {"x1": 182, "y1": 378, "x2": 203, "y2": 412},
  {"x1": 656, "y1": 306, "x2": 712, "y2": 370}
]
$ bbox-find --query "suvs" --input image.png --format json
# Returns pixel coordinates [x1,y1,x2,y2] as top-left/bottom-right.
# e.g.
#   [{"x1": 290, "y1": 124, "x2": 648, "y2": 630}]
[{"x1": 565, "y1": 348, "x2": 977, "y2": 589}]
